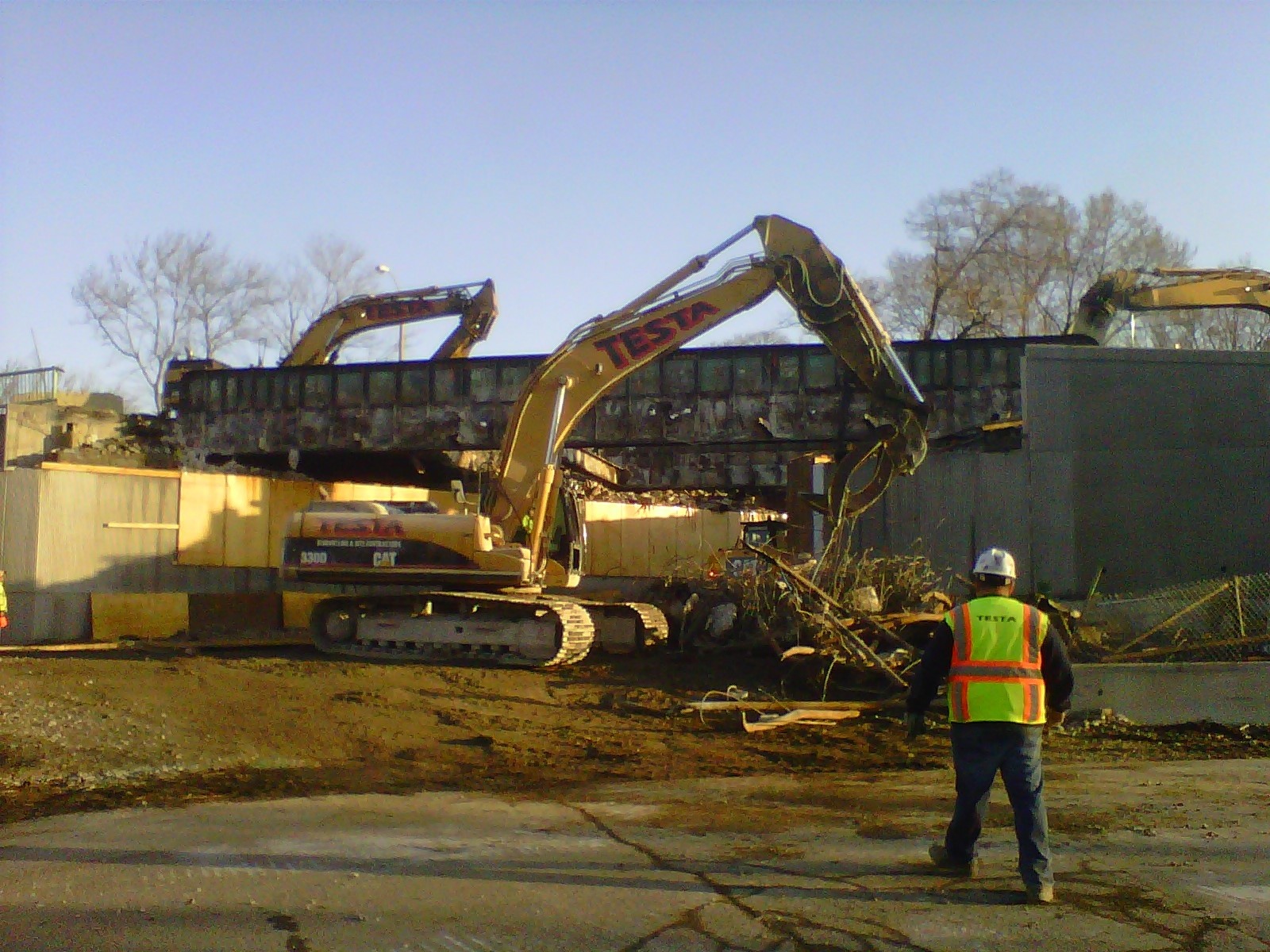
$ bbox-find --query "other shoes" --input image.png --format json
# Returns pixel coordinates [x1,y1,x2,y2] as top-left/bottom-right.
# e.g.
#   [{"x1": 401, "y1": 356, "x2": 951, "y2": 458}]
[
  {"x1": 1027, "y1": 880, "x2": 1055, "y2": 903},
  {"x1": 928, "y1": 844, "x2": 978, "y2": 878}
]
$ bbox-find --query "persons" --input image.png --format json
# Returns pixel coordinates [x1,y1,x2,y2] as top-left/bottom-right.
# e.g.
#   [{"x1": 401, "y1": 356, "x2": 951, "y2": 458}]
[
  {"x1": 521, "y1": 503, "x2": 535, "y2": 533},
  {"x1": 908, "y1": 549, "x2": 1074, "y2": 903}
]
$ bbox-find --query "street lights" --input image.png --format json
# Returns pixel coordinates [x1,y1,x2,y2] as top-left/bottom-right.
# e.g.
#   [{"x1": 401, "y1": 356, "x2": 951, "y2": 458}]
[{"x1": 376, "y1": 265, "x2": 405, "y2": 361}]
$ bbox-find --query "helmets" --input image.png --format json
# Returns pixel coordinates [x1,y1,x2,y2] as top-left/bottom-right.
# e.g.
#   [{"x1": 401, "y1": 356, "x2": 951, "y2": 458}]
[{"x1": 973, "y1": 549, "x2": 1019, "y2": 580}]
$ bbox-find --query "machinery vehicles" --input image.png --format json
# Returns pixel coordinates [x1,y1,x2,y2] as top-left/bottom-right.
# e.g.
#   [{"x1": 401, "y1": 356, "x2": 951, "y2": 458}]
[
  {"x1": 709, "y1": 517, "x2": 789, "y2": 589},
  {"x1": 124, "y1": 278, "x2": 499, "y2": 445},
  {"x1": 279, "y1": 213, "x2": 930, "y2": 672},
  {"x1": 981, "y1": 266, "x2": 1270, "y2": 455}
]
